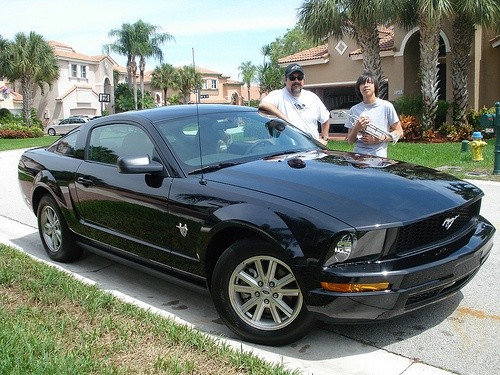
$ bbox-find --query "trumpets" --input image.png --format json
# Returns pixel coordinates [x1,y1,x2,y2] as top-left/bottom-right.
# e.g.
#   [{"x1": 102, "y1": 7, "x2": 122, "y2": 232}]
[{"x1": 340, "y1": 110, "x2": 398, "y2": 145}]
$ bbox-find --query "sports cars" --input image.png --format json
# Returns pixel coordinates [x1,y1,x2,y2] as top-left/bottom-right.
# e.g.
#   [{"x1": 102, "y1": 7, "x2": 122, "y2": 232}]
[{"x1": 17, "y1": 103, "x2": 498, "y2": 347}]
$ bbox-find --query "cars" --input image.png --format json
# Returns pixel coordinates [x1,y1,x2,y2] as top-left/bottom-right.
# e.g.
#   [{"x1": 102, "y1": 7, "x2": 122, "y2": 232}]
[{"x1": 44, "y1": 116, "x2": 93, "y2": 137}]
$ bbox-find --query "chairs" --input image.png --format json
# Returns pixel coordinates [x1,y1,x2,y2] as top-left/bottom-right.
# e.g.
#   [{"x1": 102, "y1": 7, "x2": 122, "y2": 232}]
[{"x1": 122, "y1": 132, "x2": 145, "y2": 158}]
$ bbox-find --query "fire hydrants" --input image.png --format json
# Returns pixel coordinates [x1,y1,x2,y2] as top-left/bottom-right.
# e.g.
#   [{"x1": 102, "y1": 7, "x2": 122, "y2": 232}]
[{"x1": 467, "y1": 131, "x2": 488, "y2": 163}]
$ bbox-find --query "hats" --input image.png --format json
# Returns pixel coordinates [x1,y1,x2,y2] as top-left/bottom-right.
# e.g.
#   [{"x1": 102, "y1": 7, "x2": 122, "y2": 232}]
[{"x1": 285, "y1": 64, "x2": 304, "y2": 77}]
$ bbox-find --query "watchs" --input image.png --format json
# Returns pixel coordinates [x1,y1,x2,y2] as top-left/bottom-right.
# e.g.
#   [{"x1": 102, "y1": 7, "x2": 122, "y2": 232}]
[{"x1": 322, "y1": 136, "x2": 328, "y2": 140}]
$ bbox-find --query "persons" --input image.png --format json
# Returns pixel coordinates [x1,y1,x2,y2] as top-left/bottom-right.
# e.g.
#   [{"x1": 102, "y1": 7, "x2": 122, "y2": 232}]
[
  {"x1": 259, "y1": 63, "x2": 330, "y2": 145},
  {"x1": 345, "y1": 73, "x2": 403, "y2": 159}
]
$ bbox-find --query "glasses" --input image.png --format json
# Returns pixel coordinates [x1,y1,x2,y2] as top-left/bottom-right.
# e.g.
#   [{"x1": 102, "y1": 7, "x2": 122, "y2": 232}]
[{"x1": 287, "y1": 75, "x2": 304, "y2": 81}]
